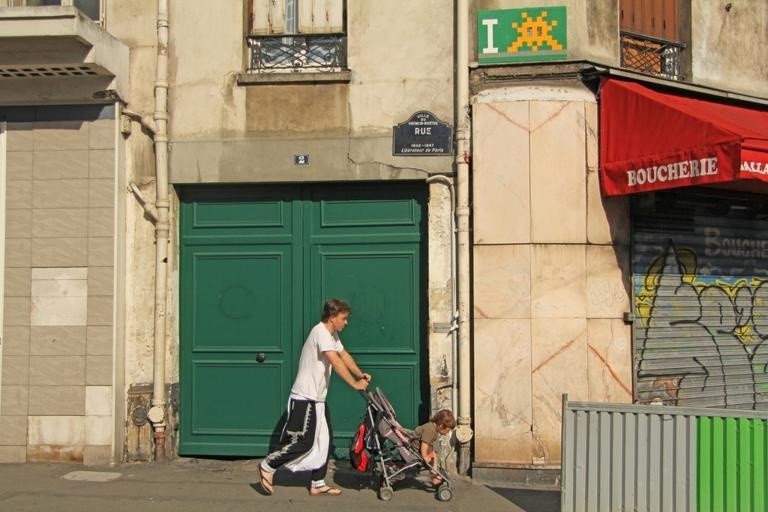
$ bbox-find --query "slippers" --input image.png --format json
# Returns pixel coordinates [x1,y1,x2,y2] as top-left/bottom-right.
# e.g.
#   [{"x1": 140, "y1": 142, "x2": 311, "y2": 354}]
[
  {"x1": 257, "y1": 461, "x2": 276, "y2": 496},
  {"x1": 310, "y1": 485, "x2": 343, "y2": 496}
]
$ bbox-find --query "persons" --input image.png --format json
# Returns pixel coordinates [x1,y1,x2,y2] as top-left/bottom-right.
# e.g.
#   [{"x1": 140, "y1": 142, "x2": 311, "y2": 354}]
[
  {"x1": 255, "y1": 296, "x2": 374, "y2": 498},
  {"x1": 410, "y1": 408, "x2": 459, "y2": 488}
]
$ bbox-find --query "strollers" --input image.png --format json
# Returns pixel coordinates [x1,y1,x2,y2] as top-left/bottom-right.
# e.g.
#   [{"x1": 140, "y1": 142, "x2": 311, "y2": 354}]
[{"x1": 355, "y1": 383, "x2": 455, "y2": 501}]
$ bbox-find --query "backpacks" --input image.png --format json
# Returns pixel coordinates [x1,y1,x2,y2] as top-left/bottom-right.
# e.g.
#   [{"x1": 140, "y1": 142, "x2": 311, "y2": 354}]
[{"x1": 347, "y1": 420, "x2": 379, "y2": 474}]
